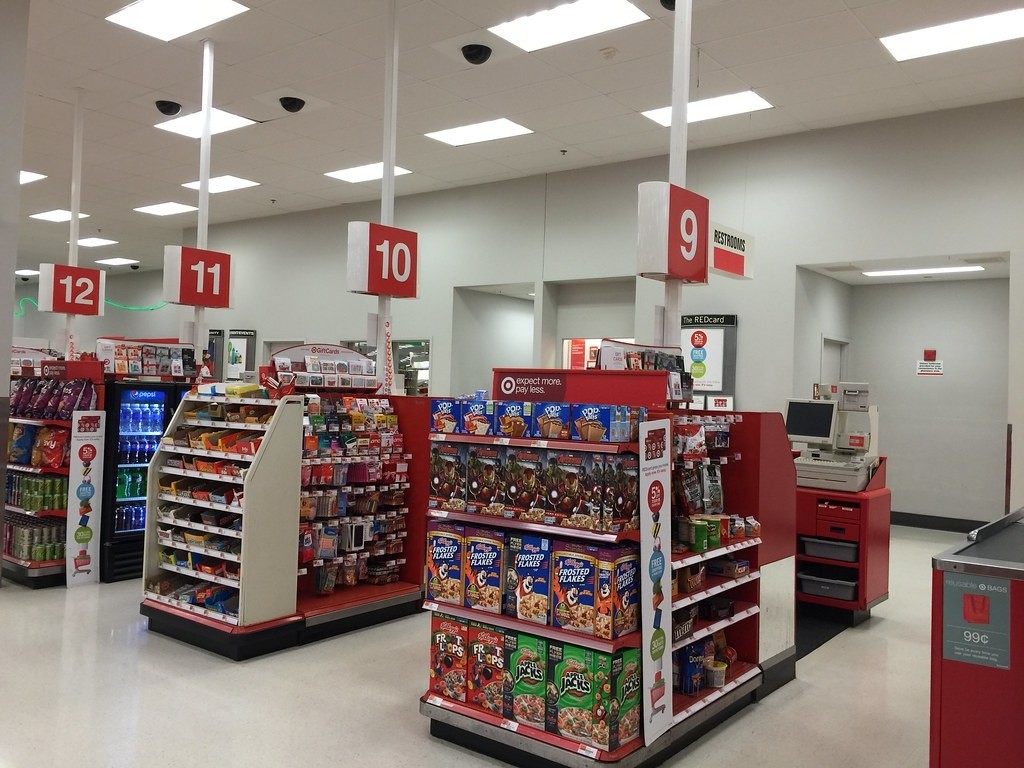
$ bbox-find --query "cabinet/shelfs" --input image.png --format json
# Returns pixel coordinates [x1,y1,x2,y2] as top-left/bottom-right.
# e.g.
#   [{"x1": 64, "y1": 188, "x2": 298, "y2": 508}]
[
  {"x1": 140, "y1": 391, "x2": 305, "y2": 662},
  {"x1": 418, "y1": 368, "x2": 671, "y2": 768},
  {"x1": 671, "y1": 537, "x2": 764, "y2": 756},
  {"x1": 2, "y1": 360, "x2": 105, "y2": 590}
]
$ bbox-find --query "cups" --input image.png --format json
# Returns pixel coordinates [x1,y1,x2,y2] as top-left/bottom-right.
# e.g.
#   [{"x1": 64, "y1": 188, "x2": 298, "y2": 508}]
[
  {"x1": 702, "y1": 661, "x2": 727, "y2": 688},
  {"x1": 716, "y1": 645, "x2": 738, "y2": 665}
]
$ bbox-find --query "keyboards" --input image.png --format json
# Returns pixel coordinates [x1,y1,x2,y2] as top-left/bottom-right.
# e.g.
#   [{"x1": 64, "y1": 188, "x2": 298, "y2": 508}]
[{"x1": 794, "y1": 457, "x2": 864, "y2": 471}]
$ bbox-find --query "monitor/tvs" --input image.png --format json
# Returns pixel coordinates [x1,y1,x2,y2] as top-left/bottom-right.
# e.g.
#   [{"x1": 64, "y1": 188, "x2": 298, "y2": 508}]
[{"x1": 784, "y1": 398, "x2": 839, "y2": 444}]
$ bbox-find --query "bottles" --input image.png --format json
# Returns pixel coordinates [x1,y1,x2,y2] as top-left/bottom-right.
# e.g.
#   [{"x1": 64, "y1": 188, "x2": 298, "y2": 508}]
[
  {"x1": 119, "y1": 403, "x2": 164, "y2": 433},
  {"x1": 115, "y1": 502, "x2": 146, "y2": 531},
  {"x1": 678, "y1": 513, "x2": 730, "y2": 552},
  {"x1": 118, "y1": 435, "x2": 160, "y2": 464},
  {"x1": 116, "y1": 467, "x2": 148, "y2": 498}
]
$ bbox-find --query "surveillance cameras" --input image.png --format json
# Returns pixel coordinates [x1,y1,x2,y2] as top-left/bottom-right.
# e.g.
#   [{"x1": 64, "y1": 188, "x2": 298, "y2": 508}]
[
  {"x1": 156, "y1": 101, "x2": 182, "y2": 116},
  {"x1": 462, "y1": 44, "x2": 492, "y2": 65},
  {"x1": 660, "y1": 0, "x2": 675, "y2": 11},
  {"x1": 131, "y1": 266, "x2": 139, "y2": 270},
  {"x1": 279, "y1": 97, "x2": 305, "y2": 113},
  {"x1": 22, "y1": 278, "x2": 29, "y2": 281}
]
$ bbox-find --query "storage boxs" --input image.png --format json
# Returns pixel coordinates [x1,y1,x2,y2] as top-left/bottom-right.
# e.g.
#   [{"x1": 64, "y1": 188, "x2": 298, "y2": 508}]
[
  {"x1": 430, "y1": 398, "x2": 649, "y2": 443},
  {"x1": 544, "y1": 450, "x2": 592, "y2": 529},
  {"x1": 545, "y1": 641, "x2": 594, "y2": 745},
  {"x1": 460, "y1": 524, "x2": 508, "y2": 615},
  {"x1": 671, "y1": 559, "x2": 750, "y2": 621},
  {"x1": 502, "y1": 631, "x2": 546, "y2": 732},
  {"x1": 672, "y1": 611, "x2": 692, "y2": 643},
  {"x1": 550, "y1": 540, "x2": 599, "y2": 636},
  {"x1": 602, "y1": 455, "x2": 640, "y2": 534},
  {"x1": 465, "y1": 444, "x2": 506, "y2": 516},
  {"x1": 595, "y1": 545, "x2": 640, "y2": 641},
  {"x1": 428, "y1": 441, "x2": 467, "y2": 512},
  {"x1": 800, "y1": 536, "x2": 857, "y2": 562},
  {"x1": 797, "y1": 571, "x2": 859, "y2": 600},
  {"x1": 591, "y1": 648, "x2": 640, "y2": 752},
  {"x1": 429, "y1": 611, "x2": 470, "y2": 703},
  {"x1": 505, "y1": 532, "x2": 554, "y2": 626},
  {"x1": 144, "y1": 356, "x2": 403, "y2": 617},
  {"x1": 503, "y1": 447, "x2": 545, "y2": 522},
  {"x1": 466, "y1": 621, "x2": 506, "y2": 715},
  {"x1": 424, "y1": 519, "x2": 465, "y2": 605}
]
